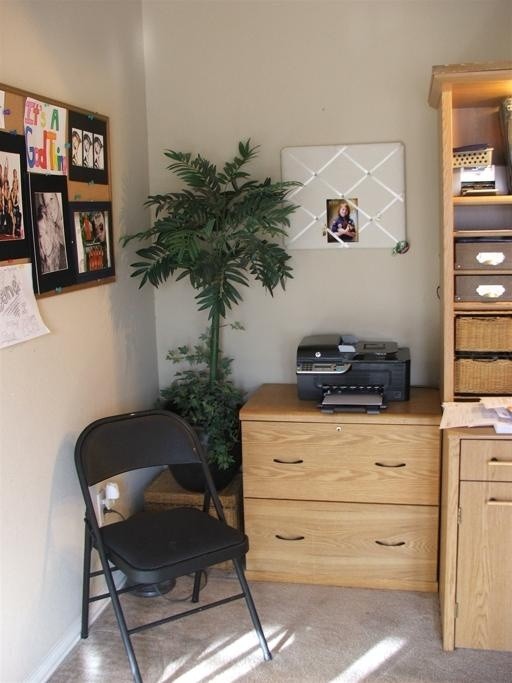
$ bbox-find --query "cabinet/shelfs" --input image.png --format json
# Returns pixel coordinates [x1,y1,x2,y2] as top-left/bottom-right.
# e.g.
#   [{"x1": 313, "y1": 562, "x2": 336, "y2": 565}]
[
  {"x1": 239, "y1": 380, "x2": 442, "y2": 594},
  {"x1": 428, "y1": 59, "x2": 512, "y2": 653}
]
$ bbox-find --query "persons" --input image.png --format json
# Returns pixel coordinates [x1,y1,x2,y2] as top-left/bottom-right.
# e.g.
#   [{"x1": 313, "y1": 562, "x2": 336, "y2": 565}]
[
  {"x1": 81, "y1": 213, "x2": 108, "y2": 268},
  {"x1": 38, "y1": 192, "x2": 66, "y2": 271},
  {"x1": 0, "y1": 157, "x2": 22, "y2": 238},
  {"x1": 331, "y1": 204, "x2": 356, "y2": 242},
  {"x1": 71, "y1": 131, "x2": 104, "y2": 170}
]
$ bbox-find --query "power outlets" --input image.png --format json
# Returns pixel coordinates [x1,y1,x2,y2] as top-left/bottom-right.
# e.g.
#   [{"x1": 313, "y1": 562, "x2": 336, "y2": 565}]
[{"x1": 97, "y1": 490, "x2": 111, "y2": 524}]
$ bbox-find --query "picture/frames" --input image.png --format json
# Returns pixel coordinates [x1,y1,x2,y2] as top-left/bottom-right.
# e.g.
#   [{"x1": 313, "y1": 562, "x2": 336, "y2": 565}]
[{"x1": 0, "y1": 80, "x2": 117, "y2": 300}]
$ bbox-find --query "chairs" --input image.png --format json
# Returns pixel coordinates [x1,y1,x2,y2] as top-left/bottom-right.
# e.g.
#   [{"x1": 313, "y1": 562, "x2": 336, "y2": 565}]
[{"x1": 74, "y1": 410, "x2": 273, "y2": 683}]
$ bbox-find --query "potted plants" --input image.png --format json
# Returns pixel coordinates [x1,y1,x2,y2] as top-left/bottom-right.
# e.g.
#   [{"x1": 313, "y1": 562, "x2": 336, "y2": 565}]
[{"x1": 117, "y1": 139, "x2": 304, "y2": 492}]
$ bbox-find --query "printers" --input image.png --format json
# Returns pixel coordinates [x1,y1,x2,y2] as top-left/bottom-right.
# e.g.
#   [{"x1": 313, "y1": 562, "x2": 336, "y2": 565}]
[{"x1": 295, "y1": 333, "x2": 412, "y2": 414}]
